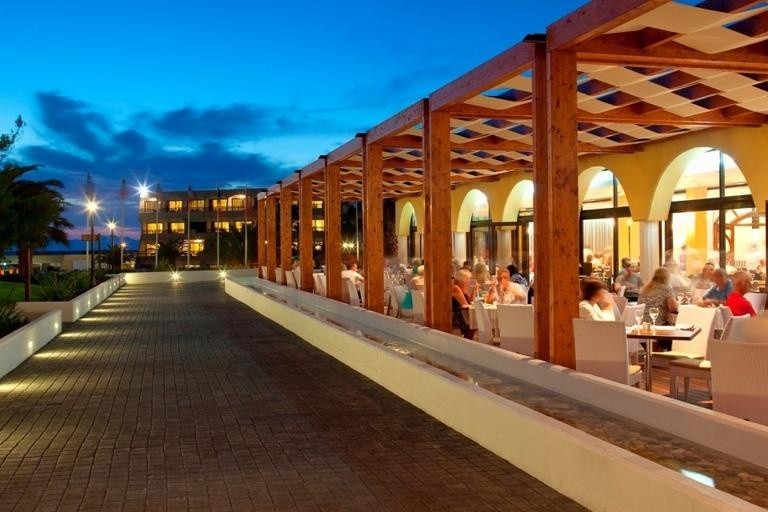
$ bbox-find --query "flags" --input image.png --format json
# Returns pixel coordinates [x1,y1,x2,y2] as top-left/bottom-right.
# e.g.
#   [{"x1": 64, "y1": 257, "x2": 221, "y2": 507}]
[
  {"x1": 120, "y1": 177, "x2": 129, "y2": 197},
  {"x1": 217, "y1": 187, "x2": 225, "y2": 220},
  {"x1": 246, "y1": 188, "x2": 254, "y2": 222},
  {"x1": 157, "y1": 183, "x2": 167, "y2": 204},
  {"x1": 188, "y1": 185, "x2": 196, "y2": 213},
  {"x1": 87, "y1": 171, "x2": 95, "y2": 194}
]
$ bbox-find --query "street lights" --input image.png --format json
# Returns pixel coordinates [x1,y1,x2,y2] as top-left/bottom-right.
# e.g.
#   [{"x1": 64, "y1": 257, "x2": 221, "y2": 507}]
[
  {"x1": 108, "y1": 222, "x2": 116, "y2": 273},
  {"x1": 87, "y1": 200, "x2": 98, "y2": 286}
]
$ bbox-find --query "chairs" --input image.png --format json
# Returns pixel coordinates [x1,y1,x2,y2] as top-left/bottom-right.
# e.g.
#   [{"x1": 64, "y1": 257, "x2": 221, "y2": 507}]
[
  {"x1": 261, "y1": 258, "x2": 427, "y2": 324},
  {"x1": 574, "y1": 259, "x2": 768, "y2": 426},
  {"x1": 452, "y1": 259, "x2": 534, "y2": 359}
]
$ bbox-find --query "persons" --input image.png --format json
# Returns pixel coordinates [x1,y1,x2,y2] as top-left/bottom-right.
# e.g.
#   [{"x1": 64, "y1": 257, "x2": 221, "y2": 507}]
[
  {"x1": 451, "y1": 253, "x2": 535, "y2": 339},
  {"x1": 391, "y1": 258, "x2": 425, "y2": 290},
  {"x1": 341, "y1": 262, "x2": 365, "y2": 303},
  {"x1": 579, "y1": 245, "x2": 767, "y2": 350}
]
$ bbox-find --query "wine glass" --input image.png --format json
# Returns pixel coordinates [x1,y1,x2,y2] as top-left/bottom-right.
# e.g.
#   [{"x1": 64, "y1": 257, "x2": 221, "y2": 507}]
[
  {"x1": 649, "y1": 308, "x2": 659, "y2": 331},
  {"x1": 677, "y1": 292, "x2": 693, "y2": 305},
  {"x1": 634, "y1": 310, "x2": 644, "y2": 331}
]
